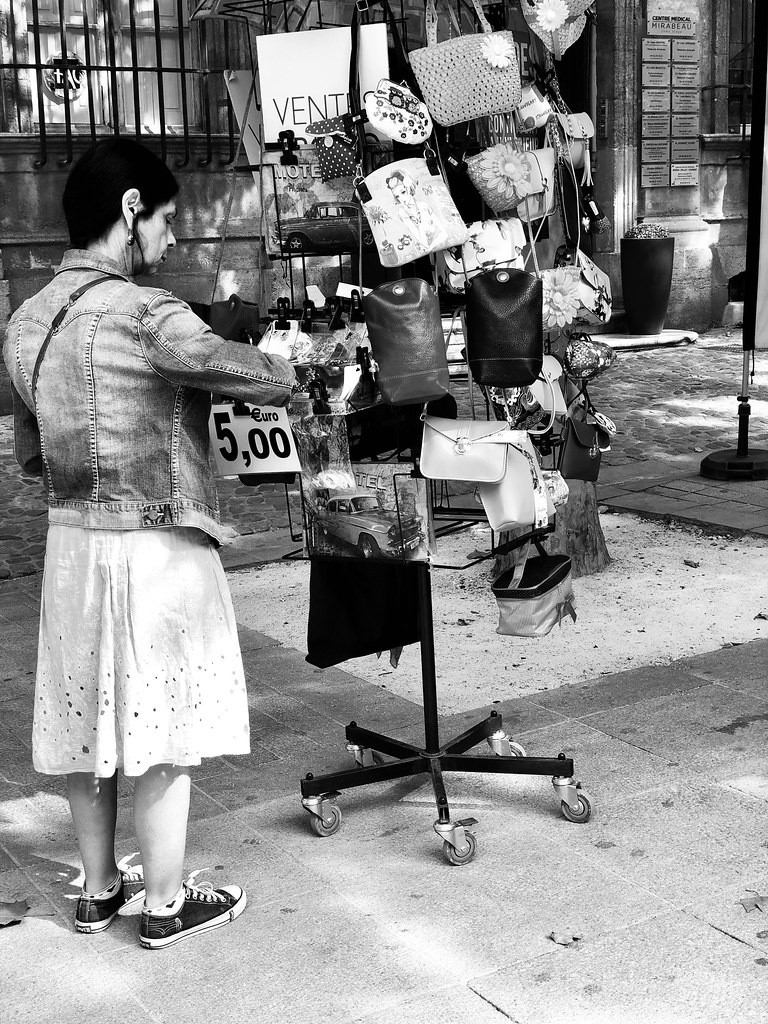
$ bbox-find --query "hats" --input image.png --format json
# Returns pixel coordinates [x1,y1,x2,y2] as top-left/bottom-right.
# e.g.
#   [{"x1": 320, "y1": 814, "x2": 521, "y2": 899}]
[{"x1": 521, "y1": 0, "x2": 597, "y2": 61}]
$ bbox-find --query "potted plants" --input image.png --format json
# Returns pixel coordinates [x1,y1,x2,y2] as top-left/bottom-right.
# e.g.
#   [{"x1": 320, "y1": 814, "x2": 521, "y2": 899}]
[{"x1": 620, "y1": 223, "x2": 675, "y2": 335}]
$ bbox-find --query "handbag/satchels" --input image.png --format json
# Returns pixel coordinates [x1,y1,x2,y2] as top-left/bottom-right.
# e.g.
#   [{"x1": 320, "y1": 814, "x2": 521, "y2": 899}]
[
  {"x1": 544, "y1": 112, "x2": 595, "y2": 186},
  {"x1": 527, "y1": 352, "x2": 569, "y2": 417},
  {"x1": 558, "y1": 369, "x2": 588, "y2": 426},
  {"x1": 564, "y1": 336, "x2": 619, "y2": 382},
  {"x1": 556, "y1": 415, "x2": 611, "y2": 484},
  {"x1": 407, "y1": 0, "x2": 524, "y2": 127},
  {"x1": 352, "y1": 148, "x2": 470, "y2": 269},
  {"x1": 428, "y1": 217, "x2": 534, "y2": 296},
  {"x1": 516, "y1": 147, "x2": 559, "y2": 224},
  {"x1": 540, "y1": 470, "x2": 569, "y2": 508},
  {"x1": 490, "y1": 537, "x2": 578, "y2": 637},
  {"x1": 462, "y1": 139, "x2": 531, "y2": 217},
  {"x1": 209, "y1": 294, "x2": 259, "y2": 345},
  {"x1": 365, "y1": 76, "x2": 435, "y2": 146},
  {"x1": 464, "y1": 268, "x2": 544, "y2": 390},
  {"x1": 357, "y1": 274, "x2": 450, "y2": 404},
  {"x1": 526, "y1": 265, "x2": 583, "y2": 333},
  {"x1": 515, "y1": 82, "x2": 555, "y2": 135},
  {"x1": 476, "y1": 430, "x2": 558, "y2": 534},
  {"x1": 419, "y1": 416, "x2": 510, "y2": 485},
  {"x1": 574, "y1": 247, "x2": 613, "y2": 327},
  {"x1": 305, "y1": 112, "x2": 361, "y2": 184}
]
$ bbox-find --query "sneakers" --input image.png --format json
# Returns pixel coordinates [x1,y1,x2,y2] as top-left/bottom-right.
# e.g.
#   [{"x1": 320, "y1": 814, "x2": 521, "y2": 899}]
[
  {"x1": 74, "y1": 864, "x2": 147, "y2": 934},
  {"x1": 137, "y1": 868, "x2": 248, "y2": 951}
]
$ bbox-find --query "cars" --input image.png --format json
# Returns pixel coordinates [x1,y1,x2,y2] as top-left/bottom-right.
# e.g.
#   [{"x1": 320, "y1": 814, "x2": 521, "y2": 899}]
[
  {"x1": 314, "y1": 494, "x2": 423, "y2": 558},
  {"x1": 271, "y1": 201, "x2": 376, "y2": 253}
]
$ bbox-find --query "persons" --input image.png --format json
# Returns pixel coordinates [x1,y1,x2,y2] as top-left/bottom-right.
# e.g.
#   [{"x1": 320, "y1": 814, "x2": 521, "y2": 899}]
[{"x1": 3, "y1": 135, "x2": 296, "y2": 951}]
